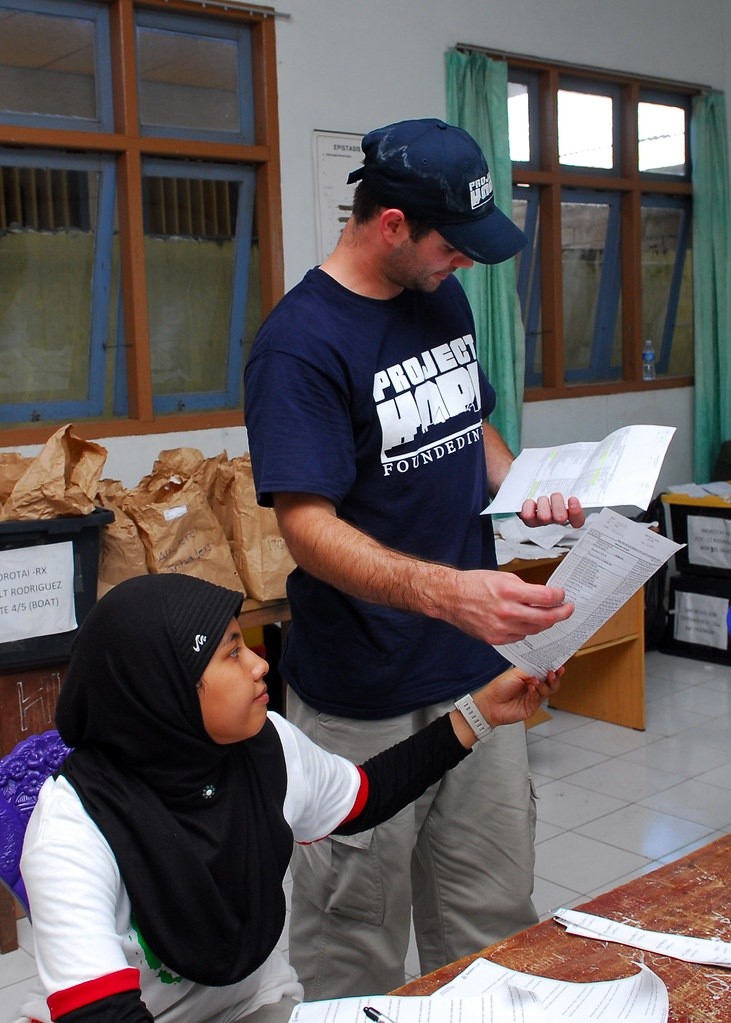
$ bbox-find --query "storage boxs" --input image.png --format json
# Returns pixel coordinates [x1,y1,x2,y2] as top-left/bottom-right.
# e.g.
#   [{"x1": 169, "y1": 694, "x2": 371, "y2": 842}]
[{"x1": 0, "y1": 507, "x2": 116, "y2": 678}]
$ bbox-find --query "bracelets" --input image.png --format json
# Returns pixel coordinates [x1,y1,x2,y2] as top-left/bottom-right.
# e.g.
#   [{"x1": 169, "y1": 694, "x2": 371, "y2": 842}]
[{"x1": 456, "y1": 694, "x2": 495, "y2": 743}]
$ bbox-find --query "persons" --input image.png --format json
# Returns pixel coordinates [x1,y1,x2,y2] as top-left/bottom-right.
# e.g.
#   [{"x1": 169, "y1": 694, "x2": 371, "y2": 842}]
[
  {"x1": 241, "y1": 119, "x2": 585, "y2": 1002},
  {"x1": 17, "y1": 575, "x2": 565, "y2": 1023}
]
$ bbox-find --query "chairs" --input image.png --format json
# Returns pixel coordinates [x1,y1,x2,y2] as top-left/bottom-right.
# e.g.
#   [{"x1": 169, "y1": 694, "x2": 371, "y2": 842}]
[{"x1": 0, "y1": 728, "x2": 76, "y2": 934}]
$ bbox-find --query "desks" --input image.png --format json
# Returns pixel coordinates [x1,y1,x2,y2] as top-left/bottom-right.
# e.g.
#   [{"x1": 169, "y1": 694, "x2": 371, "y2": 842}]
[
  {"x1": 383, "y1": 831, "x2": 731, "y2": 1023},
  {"x1": 238, "y1": 525, "x2": 659, "y2": 733}
]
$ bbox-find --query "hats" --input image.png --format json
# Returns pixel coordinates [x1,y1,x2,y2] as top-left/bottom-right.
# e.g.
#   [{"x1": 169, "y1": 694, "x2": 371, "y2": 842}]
[{"x1": 346, "y1": 118, "x2": 528, "y2": 265}]
